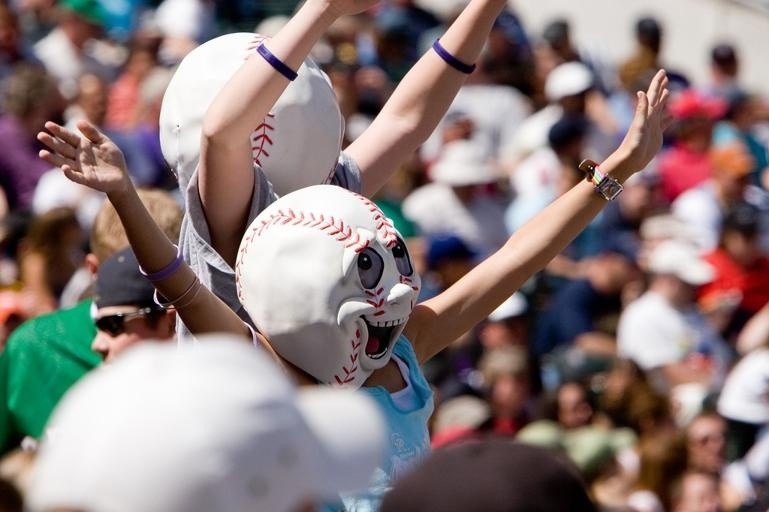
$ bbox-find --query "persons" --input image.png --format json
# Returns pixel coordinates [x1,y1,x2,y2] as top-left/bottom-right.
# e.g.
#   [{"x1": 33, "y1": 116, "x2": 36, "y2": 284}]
[{"x1": 1, "y1": 1, "x2": 769, "y2": 512}]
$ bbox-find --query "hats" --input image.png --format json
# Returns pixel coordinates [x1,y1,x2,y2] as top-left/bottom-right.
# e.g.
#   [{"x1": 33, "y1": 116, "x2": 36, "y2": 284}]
[
  {"x1": 650, "y1": 240, "x2": 716, "y2": 285},
  {"x1": 94, "y1": 246, "x2": 166, "y2": 308},
  {"x1": 378, "y1": 439, "x2": 598, "y2": 512},
  {"x1": 27, "y1": 335, "x2": 386, "y2": 512}
]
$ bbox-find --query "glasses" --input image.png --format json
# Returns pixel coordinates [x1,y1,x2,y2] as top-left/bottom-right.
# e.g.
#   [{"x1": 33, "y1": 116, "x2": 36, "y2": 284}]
[{"x1": 96, "y1": 307, "x2": 155, "y2": 337}]
